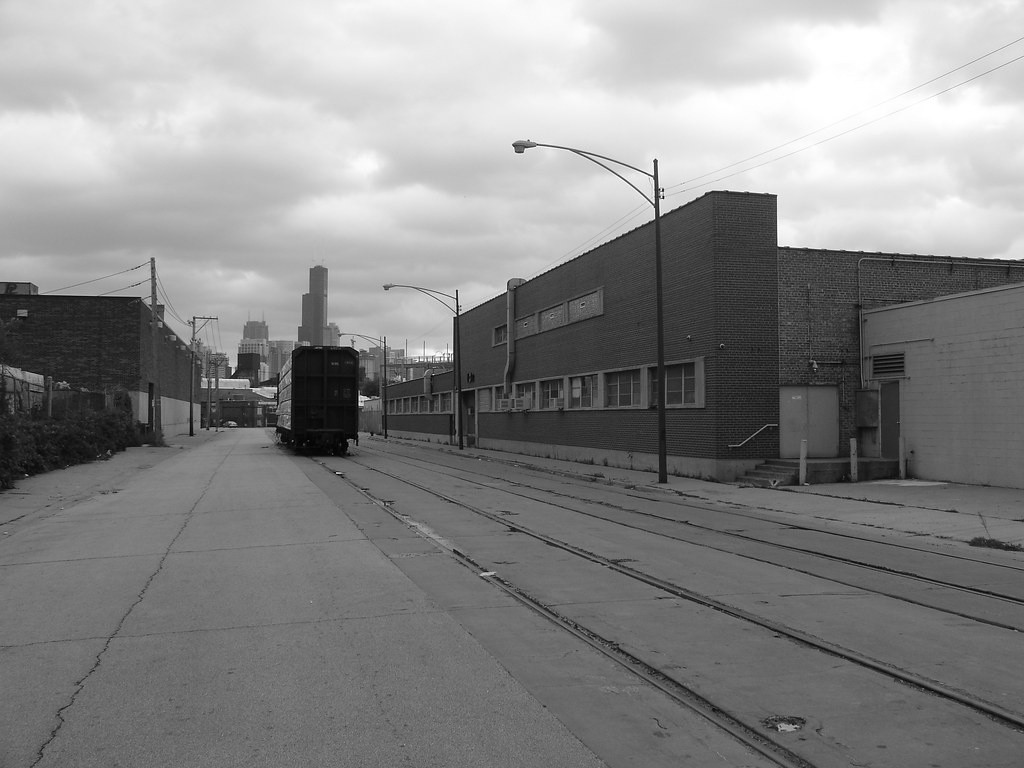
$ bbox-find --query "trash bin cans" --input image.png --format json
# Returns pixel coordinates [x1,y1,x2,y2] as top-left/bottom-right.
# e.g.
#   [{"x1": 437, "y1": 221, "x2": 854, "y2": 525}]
[{"x1": 142, "y1": 423, "x2": 151, "y2": 439}]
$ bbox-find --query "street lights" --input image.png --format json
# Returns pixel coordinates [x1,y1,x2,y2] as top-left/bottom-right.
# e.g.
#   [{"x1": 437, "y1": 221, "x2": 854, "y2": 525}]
[
  {"x1": 381, "y1": 282, "x2": 464, "y2": 451},
  {"x1": 510, "y1": 138, "x2": 668, "y2": 483},
  {"x1": 337, "y1": 332, "x2": 388, "y2": 440}
]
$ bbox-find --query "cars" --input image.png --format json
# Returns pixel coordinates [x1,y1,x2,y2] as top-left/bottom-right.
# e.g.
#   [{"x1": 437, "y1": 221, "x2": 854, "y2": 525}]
[{"x1": 222, "y1": 421, "x2": 238, "y2": 428}]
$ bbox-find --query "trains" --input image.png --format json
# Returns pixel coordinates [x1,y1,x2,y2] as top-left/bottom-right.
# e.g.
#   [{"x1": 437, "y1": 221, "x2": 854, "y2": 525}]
[{"x1": 275, "y1": 345, "x2": 358, "y2": 457}]
